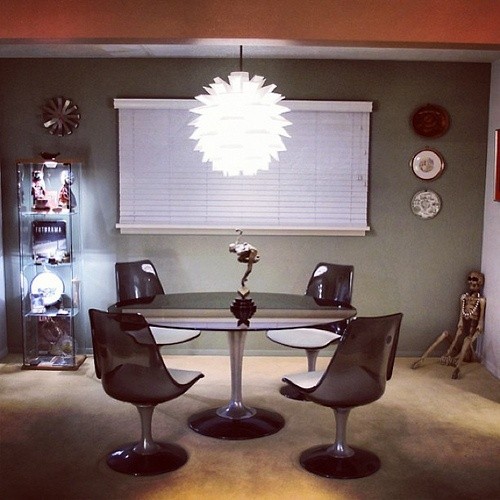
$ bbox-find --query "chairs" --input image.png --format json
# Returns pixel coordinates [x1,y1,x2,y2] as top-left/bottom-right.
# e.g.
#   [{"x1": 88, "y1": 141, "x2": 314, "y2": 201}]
[
  {"x1": 266, "y1": 262, "x2": 354, "y2": 402},
  {"x1": 88, "y1": 308, "x2": 205, "y2": 477},
  {"x1": 282, "y1": 312, "x2": 404, "y2": 479},
  {"x1": 115, "y1": 259, "x2": 200, "y2": 366}
]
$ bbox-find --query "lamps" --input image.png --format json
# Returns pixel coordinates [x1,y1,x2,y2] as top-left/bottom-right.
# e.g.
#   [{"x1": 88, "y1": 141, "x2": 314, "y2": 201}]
[{"x1": 187, "y1": 45, "x2": 293, "y2": 177}]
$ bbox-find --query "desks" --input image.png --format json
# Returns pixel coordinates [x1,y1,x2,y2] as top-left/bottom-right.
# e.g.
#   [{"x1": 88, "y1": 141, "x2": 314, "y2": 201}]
[{"x1": 107, "y1": 292, "x2": 357, "y2": 440}]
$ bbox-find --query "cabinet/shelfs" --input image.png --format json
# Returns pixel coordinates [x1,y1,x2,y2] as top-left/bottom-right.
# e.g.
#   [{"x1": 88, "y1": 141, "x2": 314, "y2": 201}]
[{"x1": 15, "y1": 157, "x2": 87, "y2": 372}]
